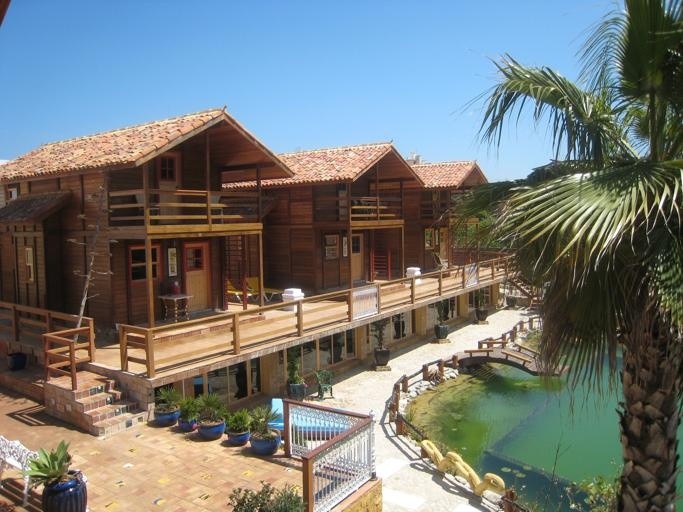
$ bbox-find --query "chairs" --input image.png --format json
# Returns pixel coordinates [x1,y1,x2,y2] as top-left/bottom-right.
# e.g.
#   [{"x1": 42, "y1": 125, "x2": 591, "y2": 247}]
[
  {"x1": 226, "y1": 279, "x2": 252, "y2": 307},
  {"x1": 431, "y1": 251, "x2": 453, "y2": 272},
  {"x1": 245, "y1": 276, "x2": 284, "y2": 305}
]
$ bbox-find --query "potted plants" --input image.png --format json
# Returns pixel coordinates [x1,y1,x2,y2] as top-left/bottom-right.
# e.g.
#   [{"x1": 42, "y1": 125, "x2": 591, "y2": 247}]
[
  {"x1": 14, "y1": 438, "x2": 88, "y2": 511},
  {"x1": 225, "y1": 408, "x2": 250, "y2": 446},
  {"x1": 152, "y1": 385, "x2": 181, "y2": 426},
  {"x1": 248, "y1": 402, "x2": 281, "y2": 455},
  {"x1": 474, "y1": 293, "x2": 488, "y2": 321},
  {"x1": 195, "y1": 392, "x2": 230, "y2": 441},
  {"x1": 368, "y1": 317, "x2": 390, "y2": 366},
  {"x1": 287, "y1": 345, "x2": 306, "y2": 401},
  {"x1": 178, "y1": 396, "x2": 197, "y2": 432},
  {"x1": 434, "y1": 300, "x2": 449, "y2": 339}
]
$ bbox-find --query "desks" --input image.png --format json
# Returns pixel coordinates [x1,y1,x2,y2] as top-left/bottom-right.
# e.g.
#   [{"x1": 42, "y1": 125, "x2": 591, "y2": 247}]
[{"x1": 157, "y1": 293, "x2": 195, "y2": 323}]
